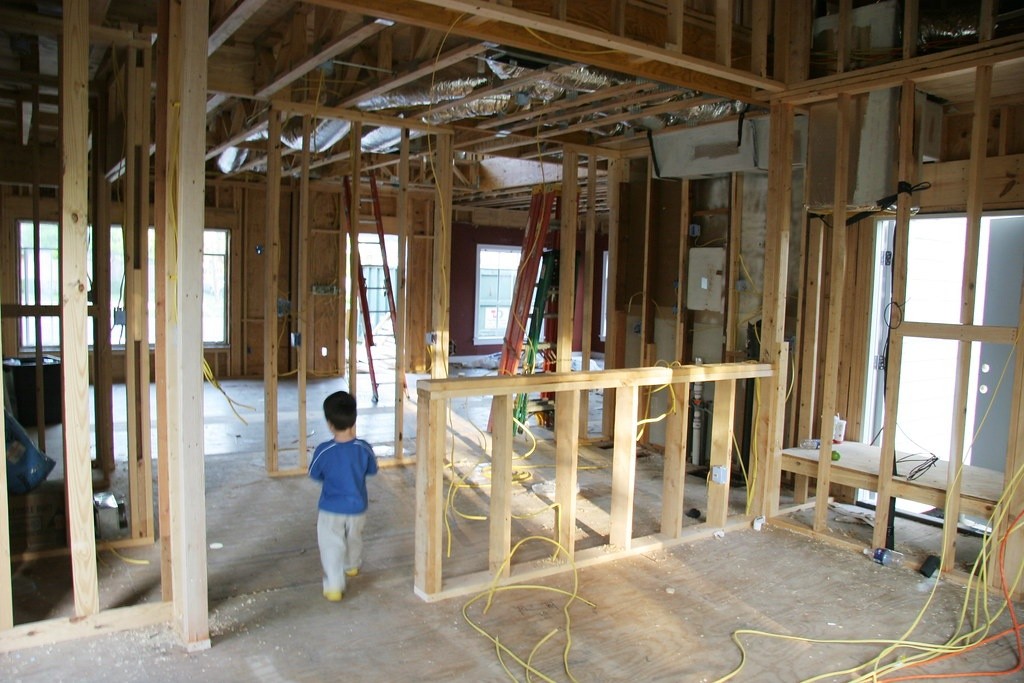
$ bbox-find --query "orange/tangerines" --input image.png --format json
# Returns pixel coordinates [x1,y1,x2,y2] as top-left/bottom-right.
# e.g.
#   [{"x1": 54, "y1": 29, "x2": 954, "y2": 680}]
[{"x1": 832, "y1": 451, "x2": 840, "y2": 460}]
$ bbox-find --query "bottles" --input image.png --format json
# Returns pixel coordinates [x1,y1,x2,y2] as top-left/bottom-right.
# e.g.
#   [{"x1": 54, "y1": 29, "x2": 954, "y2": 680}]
[
  {"x1": 800, "y1": 439, "x2": 822, "y2": 449},
  {"x1": 94, "y1": 501, "x2": 100, "y2": 539},
  {"x1": 862, "y1": 548, "x2": 905, "y2": 568},
  {"x1": 119, "y1": 494, "x2": 128, "y2": 529}
]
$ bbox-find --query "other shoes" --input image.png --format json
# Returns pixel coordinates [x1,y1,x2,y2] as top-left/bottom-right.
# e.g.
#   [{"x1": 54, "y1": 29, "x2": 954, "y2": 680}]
[
  {"x1": 345, "y1": 570, "x2": 358, "y2": 576},
  {"x1": 323, "y1": 589, "x2": 342, "y2": 602}
]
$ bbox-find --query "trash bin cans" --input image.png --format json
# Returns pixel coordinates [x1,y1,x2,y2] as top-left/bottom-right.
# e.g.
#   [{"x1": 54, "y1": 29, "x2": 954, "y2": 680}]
[{"x1": 3, "y1": 355, "x2": 62, "y2": 427}]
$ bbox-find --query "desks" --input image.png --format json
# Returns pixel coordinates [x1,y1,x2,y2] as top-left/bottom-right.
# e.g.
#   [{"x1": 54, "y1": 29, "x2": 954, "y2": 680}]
[{"x1": 780, "y1": 442, "x2": 1004, "y2": 539}]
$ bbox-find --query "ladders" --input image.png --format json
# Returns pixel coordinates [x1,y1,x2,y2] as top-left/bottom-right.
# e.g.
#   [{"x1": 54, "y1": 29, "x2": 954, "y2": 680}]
[
  {"x1": 513, "y1": 250, "x2": 578, "y2": 437},
  {"x1": 339, "y1": 174, "x2": 411, "y2": 406},
  {"x1": 485, "y1": 183, "x2": 582, "y2": 437}
]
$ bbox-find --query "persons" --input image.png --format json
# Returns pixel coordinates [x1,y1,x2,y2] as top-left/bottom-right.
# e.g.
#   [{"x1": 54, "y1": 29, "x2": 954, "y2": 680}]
[{"x1": 309, "y1": 391, "x2": 379, "y2": 600}]
